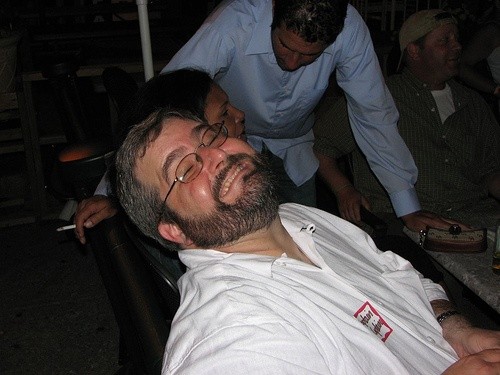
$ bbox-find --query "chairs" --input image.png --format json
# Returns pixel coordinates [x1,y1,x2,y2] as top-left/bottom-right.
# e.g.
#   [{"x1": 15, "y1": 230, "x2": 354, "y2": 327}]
[
  {"x1": 323, "y1": 153, "x2": 388, "y2": 250},
  {"x1": 100, "y1": 211, "x2": 185, "y2": 375}
]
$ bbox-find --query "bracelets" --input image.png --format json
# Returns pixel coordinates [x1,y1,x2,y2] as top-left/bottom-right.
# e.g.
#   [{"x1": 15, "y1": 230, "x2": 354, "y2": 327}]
[{"x1": 437, "y1": 310, "x2": 461, "y2": 327}]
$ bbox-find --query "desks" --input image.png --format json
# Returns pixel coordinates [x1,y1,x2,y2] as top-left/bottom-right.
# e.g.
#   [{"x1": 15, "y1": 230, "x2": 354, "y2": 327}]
[{"x1": 403, "y1": 194, "x2": 500, "y2": 326}]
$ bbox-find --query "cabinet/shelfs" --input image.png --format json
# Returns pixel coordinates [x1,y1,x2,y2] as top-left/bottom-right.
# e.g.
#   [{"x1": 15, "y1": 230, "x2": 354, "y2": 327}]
[
  {"x1": 21, "y1": 59, "x2": 169, "y2": 218},
  {"x1": 1, "y1": 107, "x2": 43, "y2": 230}
]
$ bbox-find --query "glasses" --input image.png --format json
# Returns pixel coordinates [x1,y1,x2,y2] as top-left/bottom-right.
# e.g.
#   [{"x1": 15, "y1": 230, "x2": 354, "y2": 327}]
[{"x1": 164, "y1": 120, "x2": 229, "y2": 202}]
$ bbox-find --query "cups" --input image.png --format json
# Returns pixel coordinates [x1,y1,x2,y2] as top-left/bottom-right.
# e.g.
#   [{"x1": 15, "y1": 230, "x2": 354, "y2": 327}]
[{"x1": 492, "y1": 218, "x2": 500, "y2": 274}]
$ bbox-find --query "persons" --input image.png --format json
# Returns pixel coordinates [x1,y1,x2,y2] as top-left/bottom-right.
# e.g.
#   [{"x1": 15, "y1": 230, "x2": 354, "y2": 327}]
[
  {"x1": 74, "y1": 0, "x2": 473, "y2": 246},
  {"x1": 136, "y1": 67, "x2": 248, "y2": 142},
  {"x1": 115, "y1": 108, "x2": 500, "y2": 375},
  {"x1": 456, "y1": 0, "x2": 500, "y2": 122},
  {"x1": 313, "y1": 10, "x2": 500, "y2": 223}
]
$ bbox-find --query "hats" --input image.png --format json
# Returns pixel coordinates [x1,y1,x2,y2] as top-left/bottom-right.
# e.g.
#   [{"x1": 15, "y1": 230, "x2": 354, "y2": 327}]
[{"x1": 396, "y1": 9, "x2": 460, "y2": 74}]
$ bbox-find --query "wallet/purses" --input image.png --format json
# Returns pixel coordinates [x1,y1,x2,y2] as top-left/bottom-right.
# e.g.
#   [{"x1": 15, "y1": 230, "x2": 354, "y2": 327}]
[{"x1": 422, "y1": 227, "x2": 488, "y2": 253}]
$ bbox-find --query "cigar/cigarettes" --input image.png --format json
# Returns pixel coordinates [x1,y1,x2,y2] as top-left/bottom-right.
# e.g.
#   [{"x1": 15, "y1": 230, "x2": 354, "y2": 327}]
[{"x1": 57, "y1": 224, "x2": 77, "y2": 232}]
